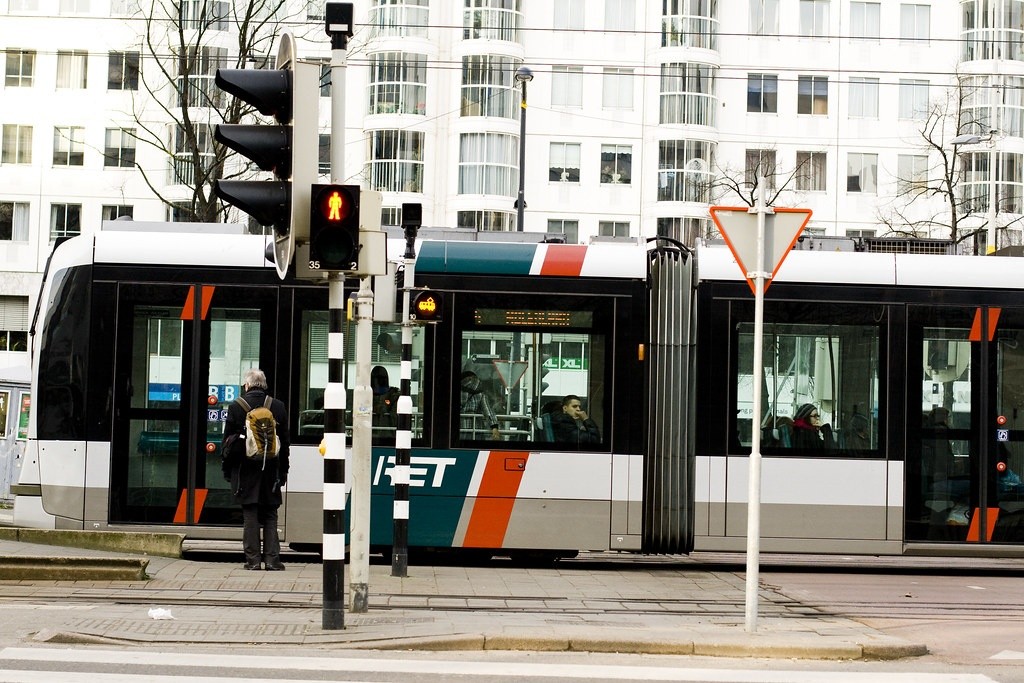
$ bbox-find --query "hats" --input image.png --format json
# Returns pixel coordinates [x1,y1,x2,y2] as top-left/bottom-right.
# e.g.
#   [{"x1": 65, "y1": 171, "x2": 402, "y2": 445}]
[{"x1": 793, "y1": 403, "x2": 817, "y2": 421}]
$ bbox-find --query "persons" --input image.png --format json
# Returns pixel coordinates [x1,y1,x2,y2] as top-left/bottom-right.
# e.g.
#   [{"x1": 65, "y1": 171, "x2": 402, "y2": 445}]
[
  {"x1": 923, "y1": 406, "x2": 971, "y2": 525},
  {"x1": 220, "y1": 369, "x2": 291, "y2": 573},
  {"x1": 303, "y1": 352, "x2": 602, "y2": 444},
  {"x1": 788, "y1": 402, "x2": 838, "y2": 450}
]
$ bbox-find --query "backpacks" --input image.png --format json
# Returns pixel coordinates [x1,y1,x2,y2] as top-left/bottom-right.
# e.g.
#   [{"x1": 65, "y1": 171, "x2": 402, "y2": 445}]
[{"x1": 236, "y1": 397, "x2": 282, "y2": 471}]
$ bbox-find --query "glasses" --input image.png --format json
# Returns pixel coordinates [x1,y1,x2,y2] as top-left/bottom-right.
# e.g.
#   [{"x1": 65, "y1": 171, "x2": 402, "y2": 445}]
[{"x1": 808, "y1": 414, "x2": 818, "y2": 420}]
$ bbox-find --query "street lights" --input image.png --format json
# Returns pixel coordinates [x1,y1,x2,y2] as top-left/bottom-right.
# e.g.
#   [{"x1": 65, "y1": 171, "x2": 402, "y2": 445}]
[{"x1": 513, "y1": 65, "x2": 534, "y2": 232}]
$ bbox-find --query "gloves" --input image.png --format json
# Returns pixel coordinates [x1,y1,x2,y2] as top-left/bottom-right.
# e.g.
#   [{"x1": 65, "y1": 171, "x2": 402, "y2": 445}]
[
  {"x1": 279, "y1": 475, "x2": 286, "y2": 487},
  {"x1": 223, "y1": 471, "x2": 231, "y2": 483}
]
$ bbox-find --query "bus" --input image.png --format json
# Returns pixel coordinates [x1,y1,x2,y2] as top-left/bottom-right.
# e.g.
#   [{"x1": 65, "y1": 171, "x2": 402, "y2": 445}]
[{"x1": 26, "y1": 213, "x2": 1024, "y2": 566}]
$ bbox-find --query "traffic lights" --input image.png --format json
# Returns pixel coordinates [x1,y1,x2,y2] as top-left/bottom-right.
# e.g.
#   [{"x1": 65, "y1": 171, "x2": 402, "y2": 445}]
[
  {"x1": 311, "y1": 185, "x2": 358, "y2": 271},
  {"x1": 213, "y1": 28, "x2": 319, "y2": 285}
]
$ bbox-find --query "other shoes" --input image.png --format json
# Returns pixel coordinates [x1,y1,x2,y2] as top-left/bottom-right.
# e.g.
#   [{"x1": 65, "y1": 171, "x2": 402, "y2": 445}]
[
  {"x1": 265, "y1": 561, "x2": 285, "y2": 570},
  {"x1": 244, "y1": 561, "x2": 261, "y2": 570}
]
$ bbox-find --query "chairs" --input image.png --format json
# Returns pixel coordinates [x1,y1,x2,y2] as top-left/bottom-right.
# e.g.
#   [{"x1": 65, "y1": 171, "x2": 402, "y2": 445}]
[
  {"x1": 777, "y1": 424, "x2": 792, "y2": 448},
  {"x1": 921, "y1": 413, "x2": 978, "y2": 499},
  {"x1": 836, "y1": 429, "x2": 848, "y2": 450},
  {"x1": 539, "y1": 413, "x2": 555, "y2": 442}
]
